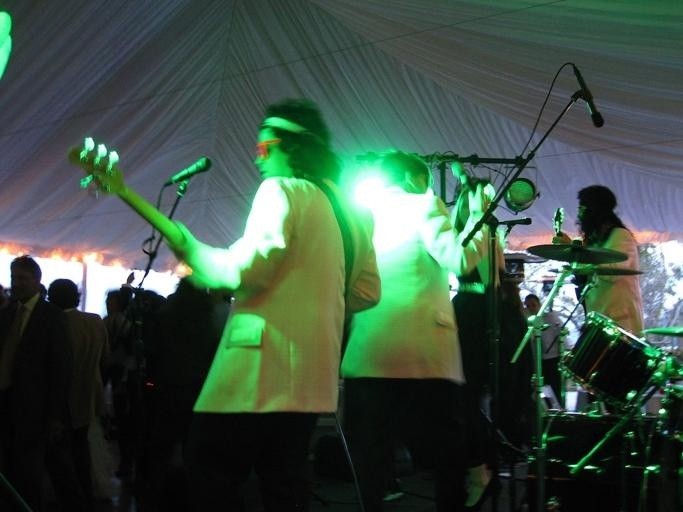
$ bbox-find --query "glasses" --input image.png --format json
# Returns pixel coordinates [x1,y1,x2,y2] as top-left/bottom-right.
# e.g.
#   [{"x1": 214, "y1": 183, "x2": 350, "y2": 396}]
[{"x1": 255, "y1": 139, "x2": 285, "y2": 160}]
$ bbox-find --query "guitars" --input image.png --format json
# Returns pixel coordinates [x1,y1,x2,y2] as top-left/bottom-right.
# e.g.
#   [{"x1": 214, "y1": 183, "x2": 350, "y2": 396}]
[{"x1": 68, "y1": 134, "x2": 185, "y2": 247}]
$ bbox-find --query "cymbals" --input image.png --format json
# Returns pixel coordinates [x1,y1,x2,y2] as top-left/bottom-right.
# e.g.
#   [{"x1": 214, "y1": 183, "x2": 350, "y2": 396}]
[
  {"x1": 639, "y1": 326, "x2": 683, "y2": 337},
  {"x1": 547, "y1": 265, "x2": 644, "y2": 276},
  {"x1": 526, "y1": 244, "x2": 628, "y2": 264},
  {"x1": 503, "y1": 248, "x2": 549, "y2": 263}
]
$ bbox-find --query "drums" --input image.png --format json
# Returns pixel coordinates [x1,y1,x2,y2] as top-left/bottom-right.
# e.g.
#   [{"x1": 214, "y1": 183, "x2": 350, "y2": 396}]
[
  {"x1": 522, "y1": 414, "x2": 663, "y2": 511},
  {"x1": 558, "y1": 311, "x2": 667, "y2": 413}
]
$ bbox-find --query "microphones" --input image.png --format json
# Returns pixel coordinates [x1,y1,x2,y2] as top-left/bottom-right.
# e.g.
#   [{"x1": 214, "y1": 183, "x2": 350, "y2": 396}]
[
  {"x1": 163, "y1": 157, "x2": 212, "y2": 183},
  {"x1": 574, "y1": 66, "x2": 604, "y2": 129},
  {"x1": 499, "y1": 217, "x2": 532, "y2": 227}
]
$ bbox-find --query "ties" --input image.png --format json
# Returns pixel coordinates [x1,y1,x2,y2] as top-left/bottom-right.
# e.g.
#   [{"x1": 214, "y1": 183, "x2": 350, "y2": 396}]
[{"x1": 1, "y1": 306, "x2": 26, "y2": 390}]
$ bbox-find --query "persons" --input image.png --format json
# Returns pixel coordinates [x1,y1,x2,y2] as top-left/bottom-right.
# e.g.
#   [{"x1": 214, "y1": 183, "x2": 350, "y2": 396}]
[{"x1": 1, "y1": 95, "x2": 646, "y2": 512}]
